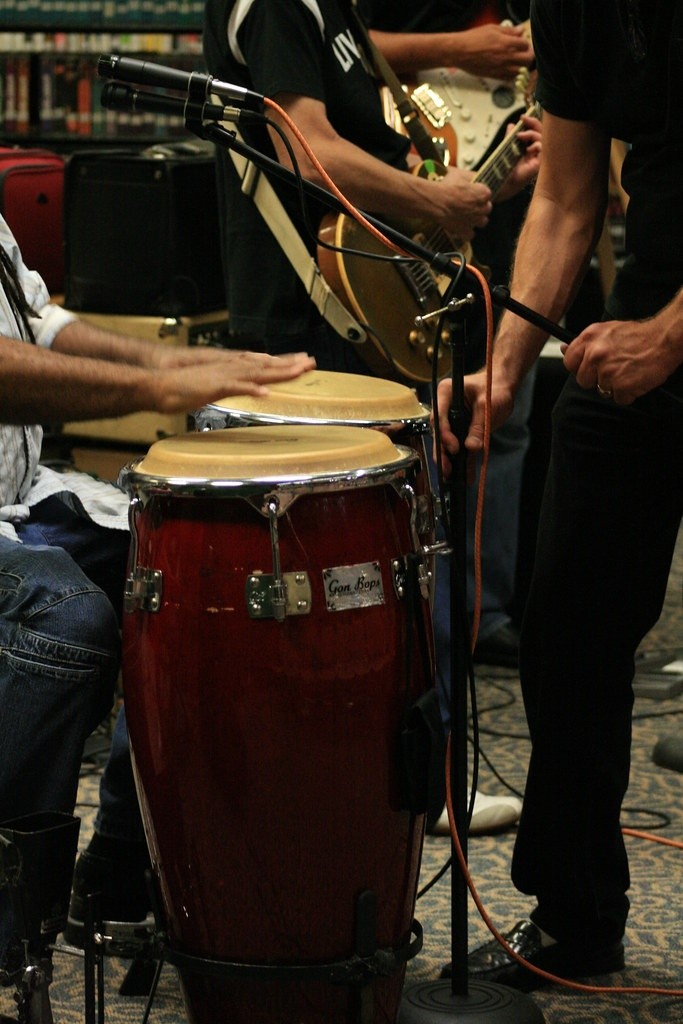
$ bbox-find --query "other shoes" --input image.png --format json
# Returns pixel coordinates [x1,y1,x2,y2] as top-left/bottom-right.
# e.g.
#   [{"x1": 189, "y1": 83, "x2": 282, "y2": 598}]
[
  {"x1": 464, "y1": 623, "x2": 520, "y2": 669},
  {"x1": 63, "y1": 852, "x2": 173, "y2": 948}
]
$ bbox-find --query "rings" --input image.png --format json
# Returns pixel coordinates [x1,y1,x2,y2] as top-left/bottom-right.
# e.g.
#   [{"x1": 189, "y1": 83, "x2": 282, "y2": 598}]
[{"x1": 597, "y1": 383, "x2": 611, "y2": 398}]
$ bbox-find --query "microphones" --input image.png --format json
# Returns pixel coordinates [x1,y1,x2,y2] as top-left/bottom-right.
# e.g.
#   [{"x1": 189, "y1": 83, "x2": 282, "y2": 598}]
[
  {"x1": 105, "y1": 82, "x2": 268, "y2": 125},
  {"x1": 98, "y1": 54, "x2": 264, "y2": 105}
]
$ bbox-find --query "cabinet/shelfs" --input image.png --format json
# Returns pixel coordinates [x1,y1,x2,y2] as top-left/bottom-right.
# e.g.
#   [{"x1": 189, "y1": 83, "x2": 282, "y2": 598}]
[{"x1": 0, "y1": 0, "x2": 206, "y2": 149}]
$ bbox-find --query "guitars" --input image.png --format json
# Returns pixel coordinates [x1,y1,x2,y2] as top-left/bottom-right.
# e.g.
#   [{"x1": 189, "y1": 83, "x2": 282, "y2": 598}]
[
  {"x1": 316, "y1": 63, "x2": 544, "y2": 387},
  {"x1": 395, "y1": 20, "x2": 534, "y2": 170}
]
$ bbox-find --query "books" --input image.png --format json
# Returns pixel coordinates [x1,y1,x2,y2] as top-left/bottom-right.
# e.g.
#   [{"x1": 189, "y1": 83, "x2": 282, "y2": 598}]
[{"x1": 0, "y1": 0, "x2": 201, "y2": 140}]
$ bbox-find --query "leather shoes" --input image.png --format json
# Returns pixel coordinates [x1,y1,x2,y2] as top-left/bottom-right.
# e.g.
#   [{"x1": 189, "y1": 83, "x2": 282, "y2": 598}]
[
  {"x1": 433, "y1": 786, "x2": 523, "y2": 836},
  {"x1": 440, "y1": 920, "x2": 625, "y2": 992}
]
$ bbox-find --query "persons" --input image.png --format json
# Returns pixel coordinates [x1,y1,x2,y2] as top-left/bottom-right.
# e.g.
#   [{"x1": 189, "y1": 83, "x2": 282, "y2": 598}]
[
  {"x1": 0, "y1": 202, "x2": 315, "y2": 982},
  {"x1": 428, "y1": 0, "x2": 683, "y2": 987},
  {"x1": 202, "y1": 0, "x2": 681, "y2": 831}
]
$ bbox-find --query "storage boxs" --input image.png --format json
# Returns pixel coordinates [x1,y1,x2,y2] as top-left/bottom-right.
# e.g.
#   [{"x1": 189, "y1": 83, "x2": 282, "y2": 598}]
[
  {"x1": 63, "y1": 149, "x2": 227, "y2": 317},
  {"x1": 51, "y1": 296, "x2": 229, "y2": 485}
]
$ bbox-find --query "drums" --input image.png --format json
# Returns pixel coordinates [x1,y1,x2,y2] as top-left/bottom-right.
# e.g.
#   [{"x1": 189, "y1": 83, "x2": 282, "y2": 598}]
[
  {"x1": 194, "y1": 370, "x2": 437, "y2": 566},
  {"x1": 122, "y1": 428, "x2": 427, "y2": 1024}
]
